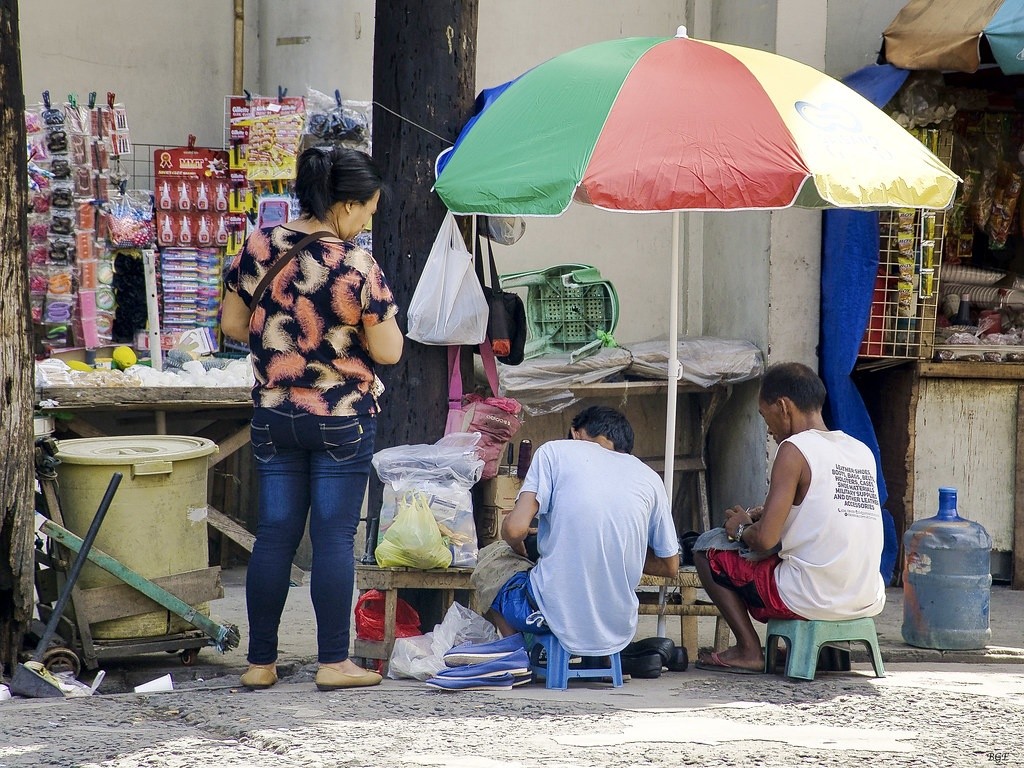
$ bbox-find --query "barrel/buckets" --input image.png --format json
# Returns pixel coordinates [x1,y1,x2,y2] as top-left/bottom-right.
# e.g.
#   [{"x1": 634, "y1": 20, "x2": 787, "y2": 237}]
[{"x1": 54, "y1": 434, "x2": 218, "y2": 636}]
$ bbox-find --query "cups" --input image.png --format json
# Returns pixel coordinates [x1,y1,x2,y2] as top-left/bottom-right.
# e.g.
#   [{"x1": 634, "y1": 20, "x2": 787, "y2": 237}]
[{"x1": 939, "y1": 263, "x2": 1024, "y2": 335}]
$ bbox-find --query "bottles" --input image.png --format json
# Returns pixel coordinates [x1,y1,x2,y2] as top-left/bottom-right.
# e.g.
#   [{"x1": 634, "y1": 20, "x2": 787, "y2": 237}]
[
  {"x1": 901, "y1": 487, "x2": 991, "y2": 650},
  {"x1": 951, "y1": 292, "x2": 972, "y2": 325}
]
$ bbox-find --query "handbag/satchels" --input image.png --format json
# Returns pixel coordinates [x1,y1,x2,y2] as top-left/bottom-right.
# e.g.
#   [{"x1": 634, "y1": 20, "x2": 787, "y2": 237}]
[
  {"x1": 487, "y1": 216, "x2": 527, "y2": 245},
  {"x1": 443, "y1": 400, "x2": 523, "y2": 480},
  {"x1": 387, "y1": 602, "x2": 500, "y2": 682},
  {"x1": 354, "y1": 589, "x2": 424, "y2": 675},
  {"x1": 405, "y1": 208, "x2": 489, "y2": 346},
  {"x1": 370, "y1": 429, "x2": 486, "y2": 571},
  {"x1": 467, "y1": 284, "x2": 527, "y2": 366}
]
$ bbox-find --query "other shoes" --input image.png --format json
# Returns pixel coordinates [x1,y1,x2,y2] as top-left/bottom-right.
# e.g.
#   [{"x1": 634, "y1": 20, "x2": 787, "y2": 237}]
[
  {"x1": 424, "y1": 632, "x2": 532, "y2": 691},
  {"x1": 240, "y1": 666, "x2": 278, "y2": 690},
  {"x1": 603, "y1": 636, "x2": 688, "y2": 679},
  {"x1": 314, "y1": 665, "x2": 382, "y2": 691}
]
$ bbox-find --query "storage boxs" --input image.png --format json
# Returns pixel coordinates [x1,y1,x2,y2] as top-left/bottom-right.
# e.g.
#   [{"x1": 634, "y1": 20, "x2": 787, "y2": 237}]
[{"x1": 475, "y1": 467, "x2": 542, "y2": 548}]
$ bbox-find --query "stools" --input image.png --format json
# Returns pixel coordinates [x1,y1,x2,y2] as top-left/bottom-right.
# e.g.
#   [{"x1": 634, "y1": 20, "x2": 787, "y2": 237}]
[
  {"x1": 763, "y1": 617, "x2": 886, "y2": 682},
  {"x1": 498, "y1": 261, "x2": 620, "y2": 365},
  {"x1": 530, "y1": 631, "x2": 626, "y2": 690}
]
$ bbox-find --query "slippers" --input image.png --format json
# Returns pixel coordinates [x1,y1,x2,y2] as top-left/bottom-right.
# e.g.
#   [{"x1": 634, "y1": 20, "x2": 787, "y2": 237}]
[
  {"x1": 775, "y1": 646, "x2": 787, "y2": 667},
  {"x1": 695, "y1": 651, "x2": 763, "y2": 674}
]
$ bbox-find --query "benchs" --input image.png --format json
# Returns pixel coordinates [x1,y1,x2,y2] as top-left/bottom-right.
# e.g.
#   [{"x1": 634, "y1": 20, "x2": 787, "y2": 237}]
[{"x1": 354, "y1": 561, "x2": 733, "y2": 681}]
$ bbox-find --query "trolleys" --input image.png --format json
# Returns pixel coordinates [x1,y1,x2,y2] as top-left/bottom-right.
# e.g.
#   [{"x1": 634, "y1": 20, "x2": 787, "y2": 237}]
[{"x1": 20, "y1": 435, "x2": 241, "y2": 678}]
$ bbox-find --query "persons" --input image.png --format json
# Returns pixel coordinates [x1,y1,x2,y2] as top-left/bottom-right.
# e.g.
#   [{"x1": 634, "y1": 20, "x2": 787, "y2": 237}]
[
  {"x1": 501, "y1": 406, "x2": 681, "y2": 638},
  {"x1": 220, "y1": 143, "x2": 406, "y2": 692},
  {"x1": 692, "y1": 362, "x2": 886, "y2": 674}
]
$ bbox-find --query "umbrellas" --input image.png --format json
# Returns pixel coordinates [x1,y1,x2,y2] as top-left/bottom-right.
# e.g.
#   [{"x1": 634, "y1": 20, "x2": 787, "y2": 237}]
[{"x1": 430, "y1": 22, "x2": 963, "y2": 639}]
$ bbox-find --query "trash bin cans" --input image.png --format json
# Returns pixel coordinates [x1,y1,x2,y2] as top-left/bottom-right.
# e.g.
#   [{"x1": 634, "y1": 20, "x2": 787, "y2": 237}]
[{"x1": 46, "y1": 432, "x2": 221, "y2": 640}]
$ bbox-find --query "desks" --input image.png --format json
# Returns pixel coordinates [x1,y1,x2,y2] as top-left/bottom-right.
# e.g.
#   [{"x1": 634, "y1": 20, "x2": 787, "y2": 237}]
[
  {"x1": 475, "y1": 358, "x2": 727, "y2": 538},
  {"x1": 32, "y1": 381, "x2": 308, "y2": 589}
]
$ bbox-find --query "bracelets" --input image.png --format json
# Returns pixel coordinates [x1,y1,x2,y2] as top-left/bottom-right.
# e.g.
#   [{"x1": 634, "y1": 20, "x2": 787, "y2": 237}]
[{"x1": 734, "y1": 523, "x2": 752, "y2": 542}]
